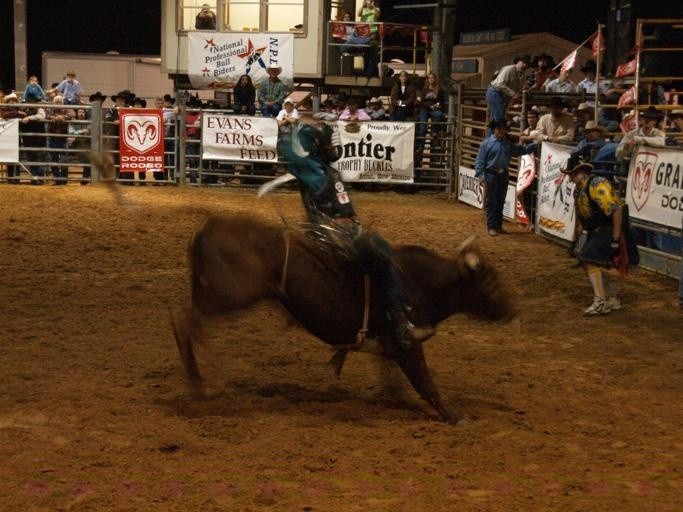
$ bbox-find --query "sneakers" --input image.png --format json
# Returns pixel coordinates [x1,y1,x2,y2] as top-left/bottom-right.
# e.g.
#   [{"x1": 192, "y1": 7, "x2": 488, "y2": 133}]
[
  {"x1": 6, "y1": 177, "x2": 243, "y2": 187},
  {"x1": 488, "y1": 225, "x2": 507, "y2": 235},
  {"x1": 583, "y1": 296, "x2": 623, "y2": 316}
]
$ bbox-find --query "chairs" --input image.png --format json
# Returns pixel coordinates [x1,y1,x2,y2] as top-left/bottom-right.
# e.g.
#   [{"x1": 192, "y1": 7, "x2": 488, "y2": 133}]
[{"x1": 330, "y1": 33, "x2": 369, "y2": 77}]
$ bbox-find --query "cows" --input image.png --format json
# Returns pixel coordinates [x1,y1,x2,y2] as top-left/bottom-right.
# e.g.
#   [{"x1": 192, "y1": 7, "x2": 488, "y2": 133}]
[{"x1": 171, "y1": 205, "x2": 519, "y2": 428}]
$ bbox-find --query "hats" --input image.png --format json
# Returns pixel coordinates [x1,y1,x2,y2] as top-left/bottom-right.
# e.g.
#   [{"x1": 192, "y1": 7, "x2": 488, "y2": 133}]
[
  {"x1": 266, "y1": 65, "x2": 282, "y2": 74},
  {"x1": 559, "y1": 157, "x2": 593, "y2": 173},
  {"x1": 572, "y1": 103, "x2": 595, "y2": 115},
  {"x1": 365, "y1": 96, "x2": 383, "y2": 107},
  {"x1": 546, "y1": 97, "x2": 568, "y2": 107},
  {"x1": 284, "y1": 98, "x2": 293, "y2": 105},
  {"x1": 579, "y1": 58, "x2": 596, "y2": 72},
  {"x1": 110, "y1": 89, "x2": 146, "y2": 108},
  {"x1": 25, "y1": 92, "x2": 41, "y2": 103},
  {"x1": 513, "y1": 54, "x2": 532, "y2": 65},
  {"x1": 578, "y1": 120, "x2": 605, "y2": 133},
  {"x1": 3, "y1": 92, "x2": 18, "y2": 102},
  {"x1": 533, "y1": 52, "x2": 553, "y2": 65},
  {"x1": 89, "y1": 91, "x2": 107, "y2": 103},
  {"x1": 639, "y1": 106, "x2": 663, "y2": 120},
  {"x1": 489, "y1": 118, "x2": 511, "y2": 130},
  {"x1": 164, "y1": 95, "x2": 176, "y2": 106}
]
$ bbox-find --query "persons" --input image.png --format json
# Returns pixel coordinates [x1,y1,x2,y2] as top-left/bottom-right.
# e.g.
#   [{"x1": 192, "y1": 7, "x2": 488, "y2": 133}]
[
  {"x1": 561, "y1": 155, "x2": 625, "y2": 318},
  {"x1": 471, "y1": 50, "x2": 683, "y2": 179},
  {"x1": 475, "y1": 119, "x2": 539, "y2": 237},
  {"x1": 280, "y1": 113, "x2": 437, "y2": 352}
]
